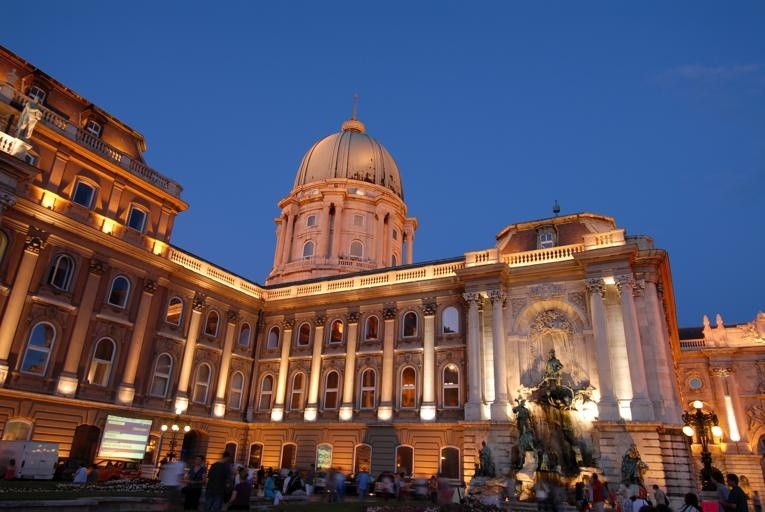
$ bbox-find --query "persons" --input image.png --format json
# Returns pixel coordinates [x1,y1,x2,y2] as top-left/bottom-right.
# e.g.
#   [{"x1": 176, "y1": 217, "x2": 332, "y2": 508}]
[{"x1": 2, "y1": 452, "x2": 764, "y2": 512}]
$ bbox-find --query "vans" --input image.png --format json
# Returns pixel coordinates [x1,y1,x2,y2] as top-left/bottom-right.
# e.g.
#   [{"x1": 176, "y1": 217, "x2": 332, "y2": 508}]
[{"x1": 90, "y1": 457, "x2": 141, "y2": 483}]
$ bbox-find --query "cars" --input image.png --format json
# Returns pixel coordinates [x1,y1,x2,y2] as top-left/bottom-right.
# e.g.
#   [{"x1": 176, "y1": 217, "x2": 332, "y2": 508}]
[{"x1": 54, "y1": 456, "x2": 87, "y2": 482}]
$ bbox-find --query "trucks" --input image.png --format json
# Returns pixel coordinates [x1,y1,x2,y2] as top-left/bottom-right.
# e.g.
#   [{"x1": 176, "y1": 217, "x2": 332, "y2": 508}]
[{"x1": 0, "y1": 439, "x2": 62, "y2": 482}]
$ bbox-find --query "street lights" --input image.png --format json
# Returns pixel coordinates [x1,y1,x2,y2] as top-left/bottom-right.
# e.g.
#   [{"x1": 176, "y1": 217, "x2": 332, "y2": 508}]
[
  {"x1": 160, "y1": 424, "x2": 190, "y2": 462},
  {"x1": 681, "y1": 398, "x2": 723, "y2": 490}
]
